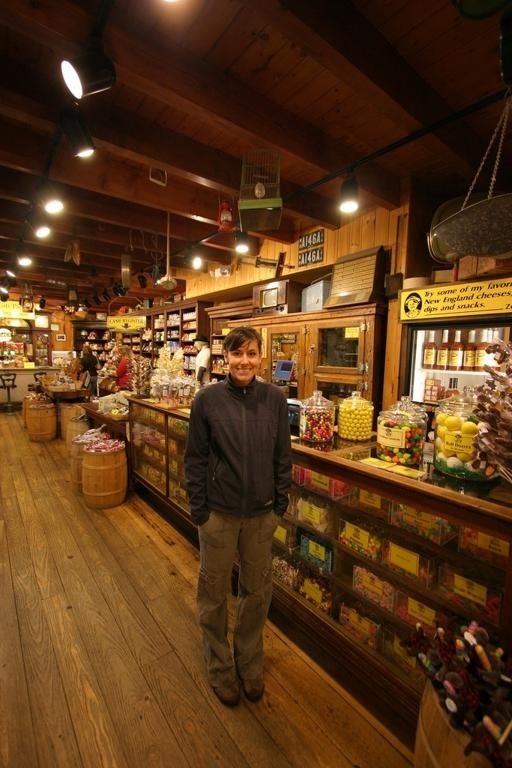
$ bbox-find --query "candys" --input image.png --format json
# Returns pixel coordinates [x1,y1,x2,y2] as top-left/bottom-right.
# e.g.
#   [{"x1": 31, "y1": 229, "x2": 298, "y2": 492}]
[
  {"x1": 337, "y1": 405, "x2": 374, "y2": 440},
  {"x1": 434, "y1": 414, "x2": 487, "y2": 472},
  {"x1": 376, "y1": 419, "x2": 424, "y2": 466},
  {"x1": 299, "y1": 411, "x2": 334, "y2": 442}
]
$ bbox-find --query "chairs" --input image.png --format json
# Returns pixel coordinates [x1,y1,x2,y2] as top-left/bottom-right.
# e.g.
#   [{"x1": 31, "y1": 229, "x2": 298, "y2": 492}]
[
  {"x1": 0, "y1": 373, "x2": 24, "y2": 413},
  {"x1": 28, "y1": 372, "x2": 47, "y2": 395}
]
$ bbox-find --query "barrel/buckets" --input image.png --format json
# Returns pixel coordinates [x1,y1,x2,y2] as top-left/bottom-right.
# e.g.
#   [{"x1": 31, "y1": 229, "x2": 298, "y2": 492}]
[
  {"x1": 81, "y1": 440, "x2": 128, "y2": 508},
  {"x1": 66, "y1": 416, "x2": 89, "y2": 457},
  {"x1": 23, "y1": 394, "x2": 30, "y2": 429},
  {"x1": 59, "y1": 402, "x2": 86, "y2": 441},
  {"x1": 71, "y1": 434, "x2": 88, "y2": 494},
  {"x1": 25, "y1": 400, "x2": 57, "y2": 442}
]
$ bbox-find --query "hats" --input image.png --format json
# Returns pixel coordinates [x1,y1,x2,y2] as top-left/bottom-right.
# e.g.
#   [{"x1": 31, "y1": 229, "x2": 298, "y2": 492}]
[{"x1": 193, "y1": 335, "x2": 210, "y2": 342}]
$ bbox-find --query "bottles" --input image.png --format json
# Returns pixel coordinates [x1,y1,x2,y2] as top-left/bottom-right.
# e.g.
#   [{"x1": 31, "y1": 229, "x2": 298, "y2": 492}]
[
  {"x1": 298, "y1": 390, "x2": 336, "y2": 442},
  {"x1": 0, "y1": 341, "x2": 17, "y2": 368},
  {"x1": 212, "y1": 360, "x2": 221, "y2": 373},
  {"x1": 422, "y1": 328, "x2": 502, "y2": 372},
  {"x1": 338, "y1": 391, "x2": 374, "y2": 441},
  {"x1": 376, "y1": 395, "x2": 429, "y2": 466},
  {"x1": 424, "y1": 377, "x2": 460, "y2": 401},
  {"x1": 433, "y1": 386, "x2": 498, "y2": 481}
]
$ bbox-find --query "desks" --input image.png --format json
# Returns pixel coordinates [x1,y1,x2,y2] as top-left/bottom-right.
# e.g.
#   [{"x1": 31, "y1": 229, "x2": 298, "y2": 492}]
[
  {"x1": 82, "y1": 400, "x2": 129, "y2": 491},
  {"x1": 39, "y1": 374, "x2": 91, "y2": 439}
]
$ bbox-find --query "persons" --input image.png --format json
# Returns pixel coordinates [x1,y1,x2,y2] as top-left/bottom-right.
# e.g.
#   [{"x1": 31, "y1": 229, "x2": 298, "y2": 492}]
[
  {"x1": 79, "y1": 346, "x2": 101, "y2": 396},
  {"x1": 184, "y1": 327, "x2": 293, "y2": 707},
  {"x1": 109, "y1": 344, "x2": 136, "y2": 391},
  {"x1": 405, "y1": 296, "x2": 421, "y2": 318},
  {"x1": 192, "y1": 335, "x2": 210, "y2": 386}
]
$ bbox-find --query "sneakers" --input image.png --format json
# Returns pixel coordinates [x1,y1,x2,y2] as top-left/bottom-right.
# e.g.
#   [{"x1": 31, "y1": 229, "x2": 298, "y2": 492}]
[{"x1": 215, "y1": 674, "x2": 264, "y2": 706}]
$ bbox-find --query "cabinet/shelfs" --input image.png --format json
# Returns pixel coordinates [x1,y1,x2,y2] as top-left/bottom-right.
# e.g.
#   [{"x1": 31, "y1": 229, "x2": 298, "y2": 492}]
[
  {"x1": 266, "y1": 324, "x2": 306, "y2": 399},
  {"x1": 309, "y1": 316, "x2": 366, "y2": 376},
  {"x1": 128, "y1": 396, "x2": 512, "y2": 724},
  {"x1": 409, "y1": 322, "x2": 512, "y2": 442},
  {"x1": 69, "y1": 297, "x2": 213, "y2": 366},
  {"x1": 308, "y1": 371, "x2": 364, "y2": 425},
  {"x1": 205, "y1": 300, "x2": 253, "y2": 382}
]
